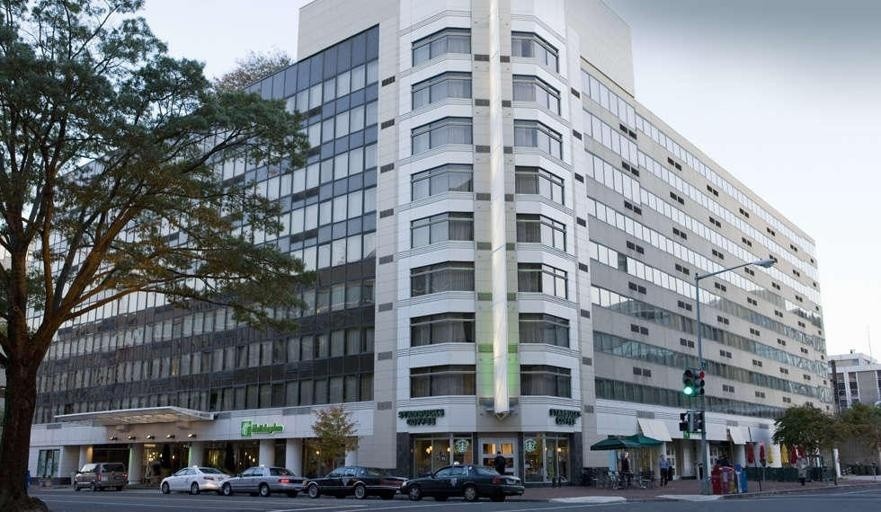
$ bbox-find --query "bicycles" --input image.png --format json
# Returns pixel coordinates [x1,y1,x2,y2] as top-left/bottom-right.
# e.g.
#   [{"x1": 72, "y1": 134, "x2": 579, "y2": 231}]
[{"x1": 609, "y1": 470, "x2": 656, "y2": 491}]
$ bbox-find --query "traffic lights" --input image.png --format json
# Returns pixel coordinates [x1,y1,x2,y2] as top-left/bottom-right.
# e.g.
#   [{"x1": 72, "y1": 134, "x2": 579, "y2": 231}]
[
  {"x1": 683, "y1": 370, "x2": 706, "y2": 397},
  {"x1": 679, "y1": 410, "x2": 704, "y2": 432}
]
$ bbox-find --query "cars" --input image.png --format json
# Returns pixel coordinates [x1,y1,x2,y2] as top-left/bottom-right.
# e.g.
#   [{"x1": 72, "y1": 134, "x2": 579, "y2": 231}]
[
  {"x1": 303, "y1": 465, "x2": 407, "y2": 499},
  {"x1": 402, "y1": 465, "x2": 524, "y2": 503},
  {"x1": 219, "y1": 466, "x2": 308, "y2": 497},
  {"x1": 160, "y1": 466, "x2": 230, "y2": 495}
]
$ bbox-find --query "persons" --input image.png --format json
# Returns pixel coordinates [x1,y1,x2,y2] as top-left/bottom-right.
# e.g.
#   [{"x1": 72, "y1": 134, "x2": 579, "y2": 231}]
[
  {"x1": 618, "y1": 450, "x2": 633, "y2": 491},
  {"x1": 795, "y1": 455, "x2": 809, "y2": 487},
  {"x1": 659, "y1": 454, "x2": 670, "y2": 487},
  {"x1": 494, "y1": 450, "x2": 506, "y2": 475}
]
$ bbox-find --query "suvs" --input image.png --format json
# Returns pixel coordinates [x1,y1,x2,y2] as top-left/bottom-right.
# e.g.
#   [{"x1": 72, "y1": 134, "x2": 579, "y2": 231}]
[{"x1": 73, "y1": 462, "x2": 127, "y2": 492}]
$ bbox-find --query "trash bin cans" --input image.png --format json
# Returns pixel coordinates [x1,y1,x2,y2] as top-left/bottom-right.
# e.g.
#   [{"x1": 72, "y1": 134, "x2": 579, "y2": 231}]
[
  {"x1": 712, "y1": 463, "x2": 746, "y2": 494},
  {"x1": 27, "y1": 470, "x2": 31, "y2": 487}
]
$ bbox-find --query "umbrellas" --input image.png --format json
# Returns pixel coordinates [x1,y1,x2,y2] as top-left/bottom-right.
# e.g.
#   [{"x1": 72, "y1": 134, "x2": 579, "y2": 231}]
[
  {"x1": 590, "y1": 436, "x2": 640, "y2": 474},
  {"x1": 626, "y1": 432, "x2": 665, "y2": 475}
]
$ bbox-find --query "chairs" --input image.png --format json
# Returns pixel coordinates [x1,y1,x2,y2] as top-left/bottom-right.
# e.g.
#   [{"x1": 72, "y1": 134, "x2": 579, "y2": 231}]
[{"x1": 605, "y1": 470, "x2": 654, "y2": 490}]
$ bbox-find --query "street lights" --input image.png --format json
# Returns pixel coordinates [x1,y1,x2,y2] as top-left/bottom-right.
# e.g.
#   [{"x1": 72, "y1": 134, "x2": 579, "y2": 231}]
[{"x1": 695, "y1": 259, "x2": 774, "y2": 493}]
